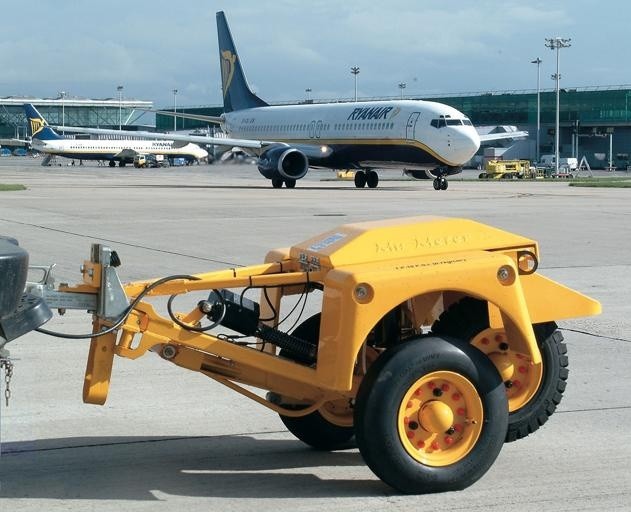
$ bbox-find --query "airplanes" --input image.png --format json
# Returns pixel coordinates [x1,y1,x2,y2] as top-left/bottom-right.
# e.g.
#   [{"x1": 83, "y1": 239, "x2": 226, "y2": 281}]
[
  {"x1": 42, "y1": 11, "x2": 529, "y2": 190},
  {"x1": 11, "y1": 104, "x2": 209, "y2": 168}
]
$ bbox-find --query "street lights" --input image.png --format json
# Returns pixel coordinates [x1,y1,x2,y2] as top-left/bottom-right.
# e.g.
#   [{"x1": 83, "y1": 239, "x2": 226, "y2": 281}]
[
  {"x1": 398, "y1": 82, "x2": 407, "y2": 100},
  {"x1": 350, "y1": 67, "x2": 360, "y2": 102},
  {"x1": 59, "y1": 92, "x2": 67, "y2": 136},
  {"x1": 305, "y1": 88, "x2": 312, "y2": 100},
  {"x1": 531, "y1": 58, "x2": 544, "y2": 160},
  {"x1": 117, "y1": 85, "x2": 124, "y2": 130},
  {"x1": 544, "y1": 36, "x2": 573, "y2": 179},
  {"x1": 172, "y1": 90, "x2": 179, "y2": 132}
]
